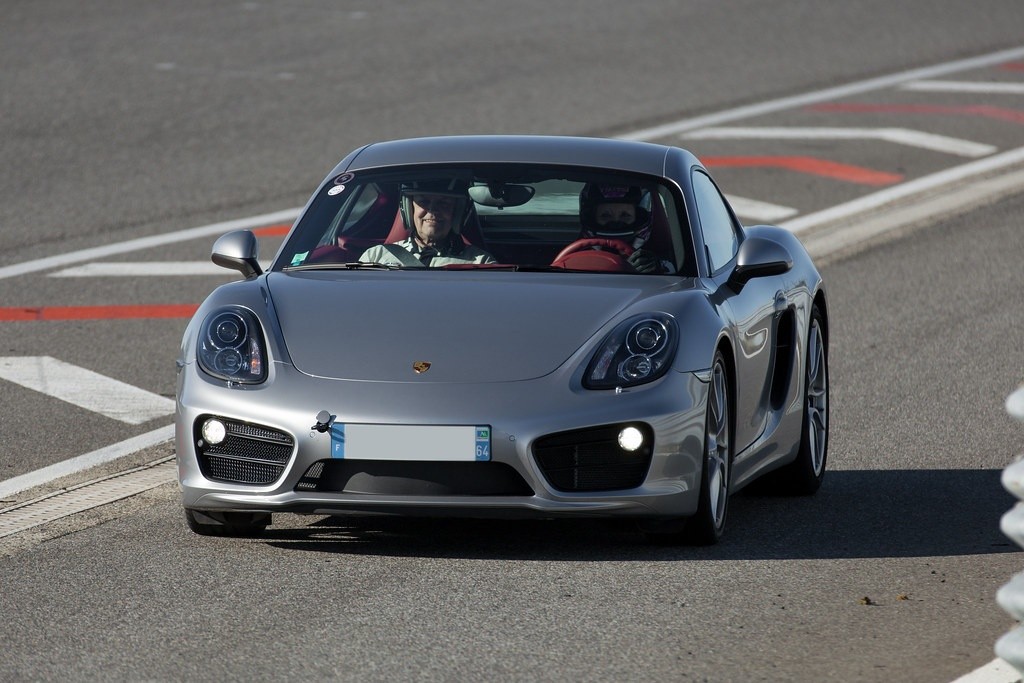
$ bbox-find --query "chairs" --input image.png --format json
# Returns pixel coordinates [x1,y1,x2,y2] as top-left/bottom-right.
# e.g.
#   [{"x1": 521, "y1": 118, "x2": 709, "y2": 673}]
[
  {"x1": 382, "y1": 194, "x2": 488, "y2": 246},
  {"x1": 640, "y1": 188, "x2": 678, "y2": 272}
]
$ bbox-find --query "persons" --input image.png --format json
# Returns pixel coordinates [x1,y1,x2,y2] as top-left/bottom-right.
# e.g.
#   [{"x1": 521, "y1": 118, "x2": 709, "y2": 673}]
[
  {"x1": 574, "y1": 177, "x2": 677, "y2": 275},
  {"x1": 358, "y1": 178, "x2": 500, "y2": 269}
]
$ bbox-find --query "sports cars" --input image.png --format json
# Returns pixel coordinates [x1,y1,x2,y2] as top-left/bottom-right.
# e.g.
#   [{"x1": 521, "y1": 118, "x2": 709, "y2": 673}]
[{"x1": 171, "y1": 135, "x2": 832, "y2": 548}]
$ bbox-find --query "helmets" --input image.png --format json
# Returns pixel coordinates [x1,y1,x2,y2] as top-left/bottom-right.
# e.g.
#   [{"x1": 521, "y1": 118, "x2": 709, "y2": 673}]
[
  {"x1": 398, "y1": 174, "x2": 475, "y2": 235},
  {"x1": 578, "y1": 183, "x2": 654, "y2": 249}
]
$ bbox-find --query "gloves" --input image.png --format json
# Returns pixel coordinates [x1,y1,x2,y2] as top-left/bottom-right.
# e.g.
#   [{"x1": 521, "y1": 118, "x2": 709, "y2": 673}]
[{"x1": 627, "y1": 248, "x2": 665, "y2": 276}]
